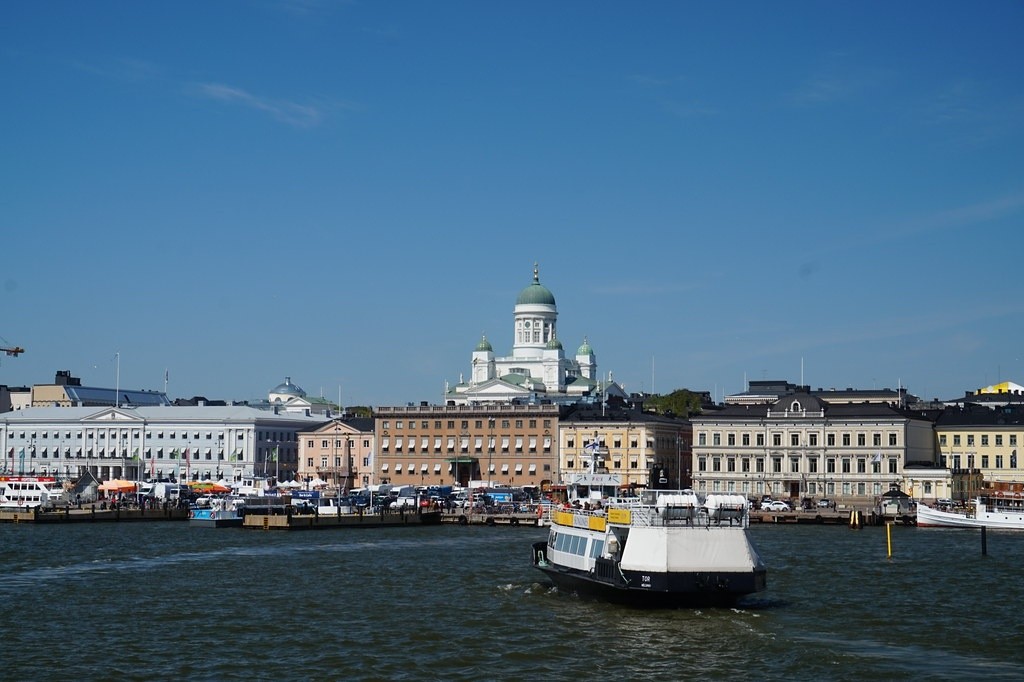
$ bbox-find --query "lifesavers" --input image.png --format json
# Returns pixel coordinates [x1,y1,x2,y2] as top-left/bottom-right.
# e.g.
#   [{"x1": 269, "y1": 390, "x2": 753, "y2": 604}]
[
  {"x1": 17, "y1": 499, "x2": 24, "y2": 505},
  {"x1": 510, "y1": 517, "x2": 519, "y2": 526},
  {"x1": 210, "y1": 512, "x2": 215, "y2": 519},
  {"x1": 458, "y1": 515, "x2": 467, "y2": 525},
  {"x1": 538, "y1": 505, "x2": 542, "y2": 518},
  {"x1": 486, "y1": 517, "x2": 494, "y2": 526}
]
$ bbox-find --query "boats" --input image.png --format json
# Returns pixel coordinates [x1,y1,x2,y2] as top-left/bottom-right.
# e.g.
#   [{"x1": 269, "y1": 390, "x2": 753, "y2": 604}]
[
  {"x1": 871, "y1": 482, "x2": 917, "y2": 527},
  {"x1": 528, "y1": 485, "x2": 769, "y2": 600},
  {"x1": 0, "y1": 480, "x2": 65, "y2": 509},
  {"x1": 917, "y1": 454, "x2": 1024, "y2": 531},
  {"x1": 189, "y1": 493, "x2": 246, "y2": 527}
]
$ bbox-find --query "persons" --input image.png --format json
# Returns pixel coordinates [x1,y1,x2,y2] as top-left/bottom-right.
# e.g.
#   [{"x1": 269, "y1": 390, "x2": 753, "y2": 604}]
[
  {"x1": 99, "y1": 489, "x2": 181, "y2": 511},
  {"x1": 833, "y1": 500, "x2": 837, "y2": 513},
  {"x1": 428, "y1": 498, "x2": 459, "y2": 514},
  {"x1": 559, "y1": 494, "x2": 614, "y2": 517},
  {"x1": 351, "y1": 497, "x2": 357, "y2": 514},
  {"x1": 617, "y1": 488, "x2": 628, "y2": 497},
  {"x1": 77, "y1": 493, "x2": 81, "y2": 504}
]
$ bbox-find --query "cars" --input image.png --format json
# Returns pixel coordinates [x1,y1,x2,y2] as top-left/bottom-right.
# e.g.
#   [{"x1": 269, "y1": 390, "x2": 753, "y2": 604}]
[
  {"x1": 935, "y1": 498, "x2": 960, "y2": 507},
  {"x1": 761, "y1": 491, "x2": 816, "y2": 513},
  {"x1": 341, "y1": 484, "x2": 533, "y2": 509},
  {"x1": 815, "y1": 498, "x2": 835, "y2": 508}
]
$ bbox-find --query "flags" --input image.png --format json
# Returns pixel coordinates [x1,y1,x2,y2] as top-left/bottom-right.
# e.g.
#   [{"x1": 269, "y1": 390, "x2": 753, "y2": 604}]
[
  {"x1": 230, "y1": 450, "x2": 236, "y2": 462},
  {"x1": 272, "y1": 448, "x2": 277, "y2": 461},
  {"x1": 186, "y1": 447, "x2": 190, "y2": 468},
  {"x1": 151, "y1": 456, "x2": 155, "y2": 476}
]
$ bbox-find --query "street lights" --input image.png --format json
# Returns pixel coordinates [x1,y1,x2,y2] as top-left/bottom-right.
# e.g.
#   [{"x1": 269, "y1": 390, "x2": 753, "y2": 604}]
[
  {"x1": 487, "y1": 416, "x2": 496, "y2": 489},
  {"x1": 677, "y1": 434, "x2": 685, "y2": 492}
]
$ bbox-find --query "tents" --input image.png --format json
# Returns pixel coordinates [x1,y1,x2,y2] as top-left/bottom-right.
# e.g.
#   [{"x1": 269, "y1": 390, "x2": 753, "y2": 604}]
[
  {"x1": 278, "y1": 478, "x2": 329, "y2": 490},
  {"x1": 98, "y1": 479, "x2": 134, "y2": 495},
  {"x1": 188, "y1": 478, "x2": 240, "y2": 494}
]
